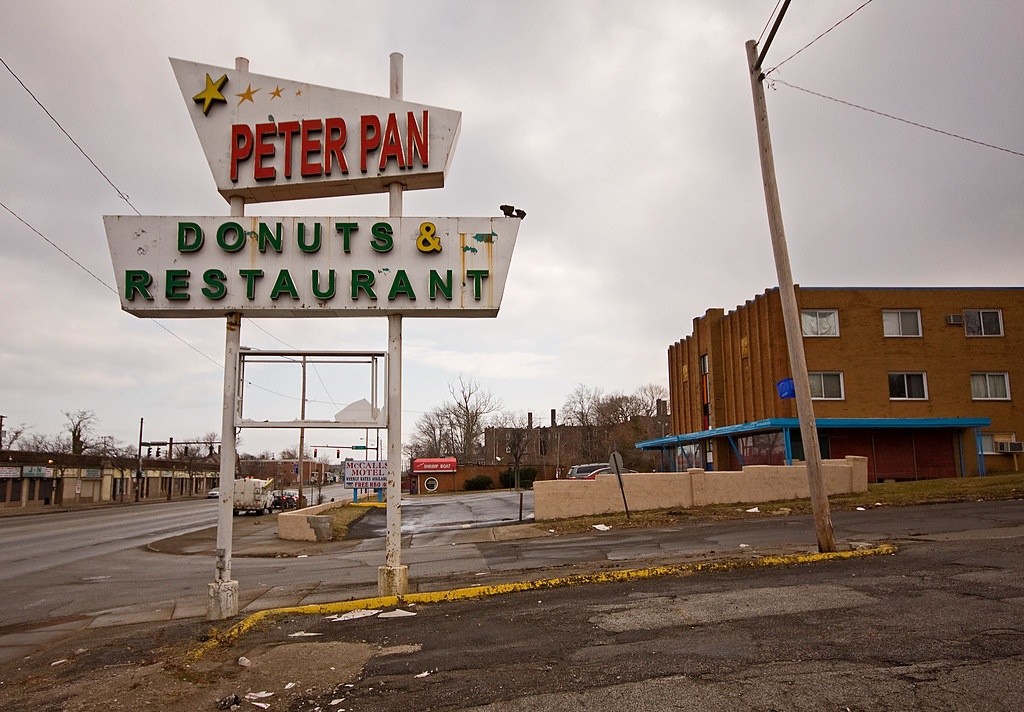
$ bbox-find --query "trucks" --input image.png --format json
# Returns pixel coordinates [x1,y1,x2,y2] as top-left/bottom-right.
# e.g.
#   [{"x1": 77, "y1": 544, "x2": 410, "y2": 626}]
[{"x1": 233, "y1": 476, "x2": 275, "y2": 515}]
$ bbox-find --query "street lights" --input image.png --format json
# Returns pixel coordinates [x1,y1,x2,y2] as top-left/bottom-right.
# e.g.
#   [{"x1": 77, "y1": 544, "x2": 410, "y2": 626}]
[{"x1": 48, "y1": 459, "x2": 64, "y2": 507}]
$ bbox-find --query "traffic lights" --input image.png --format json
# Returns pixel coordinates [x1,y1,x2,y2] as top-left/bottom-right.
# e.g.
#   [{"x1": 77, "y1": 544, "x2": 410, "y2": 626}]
[
  {"x1": 147, "y1": 447, "x2": 152, "y2": 458},
  {"x1": 314, "y1": 449, "x2": 317, "y2": 457},
  {"x1": 156, "y1": 448, "x2": 161, "y2": 457},
  {"x1": 209, "y1": 445, "x2": 214, "y2": 455},
  {"x1": 184, "y1": 446, "x2": 189, "y2": 456},
  {"x1": 337, "y1": 450, "x2": 340, "y2": 458}
]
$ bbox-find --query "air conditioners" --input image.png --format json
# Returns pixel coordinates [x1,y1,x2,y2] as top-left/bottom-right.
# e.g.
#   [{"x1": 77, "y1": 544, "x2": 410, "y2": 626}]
[
  {"x1": 945, "y1": 314, "x2": 963, "y2": 325},
  {"x1": 994, "y1": 441, "x2": 1023, "y2": 453}
]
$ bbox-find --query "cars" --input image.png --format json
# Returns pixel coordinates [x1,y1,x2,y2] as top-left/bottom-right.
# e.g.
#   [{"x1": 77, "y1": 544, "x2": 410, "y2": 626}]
[
  {"x1": 271, "y1": 490, "x2": 295, "y2": 509},
  {"x1": 208, "y1": 487, "x2": 220, "y2": 499},
  {"x1": 581, "y1": 467, "x2": 638, "y2": 480},
  {"x1": 288, "y1": 491, "x2": 307, "y2": 507}
]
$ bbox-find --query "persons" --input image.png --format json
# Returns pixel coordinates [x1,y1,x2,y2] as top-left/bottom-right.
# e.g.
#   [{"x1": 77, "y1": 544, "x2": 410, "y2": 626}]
[{"x1": 283, "y1": 492, "x2": 292, "y2": 497}]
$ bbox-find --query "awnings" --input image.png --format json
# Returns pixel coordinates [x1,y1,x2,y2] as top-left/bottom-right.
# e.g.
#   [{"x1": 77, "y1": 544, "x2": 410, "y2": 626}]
[{"x1": 636, "y1": 418, "x2": 991, "y2": 451}]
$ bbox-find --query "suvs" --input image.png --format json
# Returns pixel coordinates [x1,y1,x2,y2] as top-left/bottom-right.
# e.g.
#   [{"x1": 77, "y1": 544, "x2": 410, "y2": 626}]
[{"x1": 565, "y1": 462, "x2": 623, "y2": 479}]
